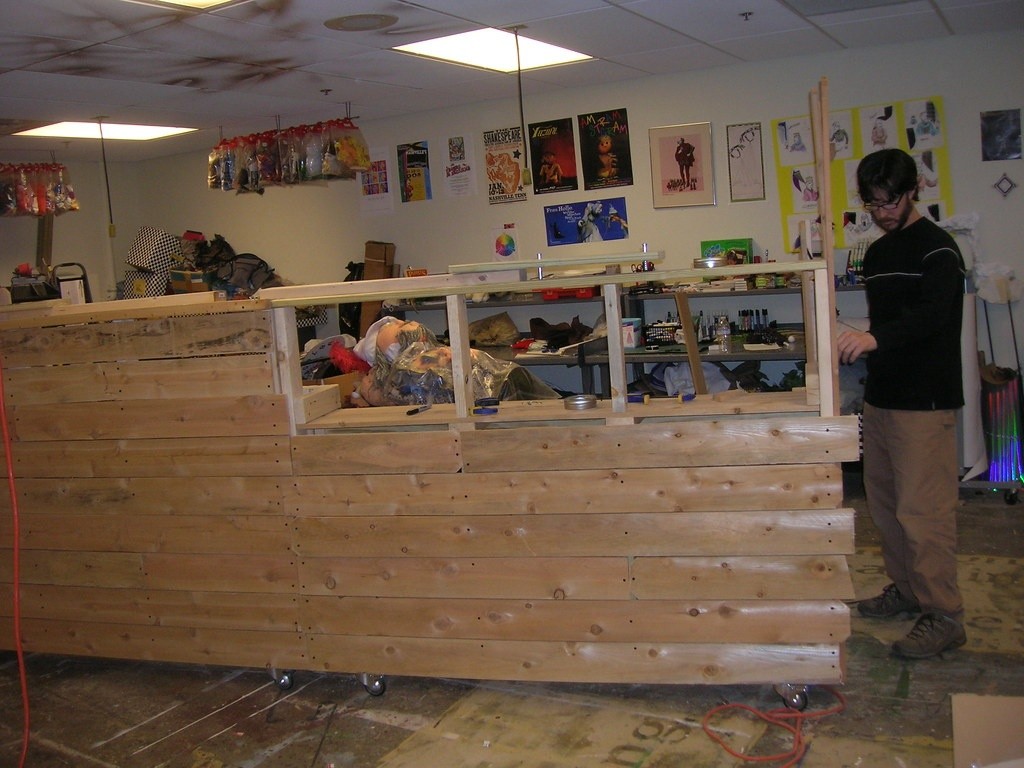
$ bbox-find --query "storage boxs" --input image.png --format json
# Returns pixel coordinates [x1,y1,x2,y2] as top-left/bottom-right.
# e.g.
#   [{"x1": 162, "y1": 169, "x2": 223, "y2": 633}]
[
  {"x1": 123, "y1": 271, "x2": 172, "y2": 300},
  {"x1": 124, "y1": 226, "x2": 182, "y2": 280}
]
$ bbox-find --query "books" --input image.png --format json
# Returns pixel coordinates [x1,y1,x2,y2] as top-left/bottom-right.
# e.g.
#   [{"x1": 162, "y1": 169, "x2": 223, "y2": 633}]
[{"x1": 703, "y1": 277, "x2": 752, "y2": 292}]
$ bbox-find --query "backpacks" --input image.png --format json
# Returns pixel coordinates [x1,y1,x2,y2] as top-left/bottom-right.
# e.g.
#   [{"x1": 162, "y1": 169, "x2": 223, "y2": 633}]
[{"x1": 217, "y1": 253, "x2": 275, "y2": 296}]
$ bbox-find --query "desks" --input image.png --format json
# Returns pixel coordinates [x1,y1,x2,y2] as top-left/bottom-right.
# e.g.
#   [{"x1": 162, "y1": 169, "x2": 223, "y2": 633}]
[
  {"x1": 587, "y1": 323, "x2": 808, "y2": 396},
  {"x1": 434, "y1": 332, "x2": 601, "y2": 395}
]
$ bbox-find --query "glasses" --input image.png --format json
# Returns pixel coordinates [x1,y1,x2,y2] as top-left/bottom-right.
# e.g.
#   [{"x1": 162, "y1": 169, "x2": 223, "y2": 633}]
[{"x1": 864, "y1": 192, "x2": 906, "y2": 211}]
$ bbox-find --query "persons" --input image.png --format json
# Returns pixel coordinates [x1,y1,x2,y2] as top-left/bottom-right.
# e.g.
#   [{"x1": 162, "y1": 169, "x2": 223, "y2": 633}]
[
  {"x1": 834, "y1": 149, "x2": 968, "y2": 659},
  {"x1": 675, "y1": 138, "x2": 695, "y2": 187}
]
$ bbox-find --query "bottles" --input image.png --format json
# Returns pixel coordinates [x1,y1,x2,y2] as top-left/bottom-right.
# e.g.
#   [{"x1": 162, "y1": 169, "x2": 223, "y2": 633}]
[
  {"x1": 718, "y1": 316, "x2": 731, "y2": 353},
  {"x1": 749, "y1": 255, "x2": 762, "y2": 289}
]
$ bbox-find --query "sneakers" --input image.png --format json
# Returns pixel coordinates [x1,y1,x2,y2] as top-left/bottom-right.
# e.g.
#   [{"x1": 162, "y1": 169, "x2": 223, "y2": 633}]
[
  {"x1": 858, "y1": 582, "x2": 919, "y2": 618},
  {"x1": 891, "y1": 612, "x2": 967, "y2": 658}
]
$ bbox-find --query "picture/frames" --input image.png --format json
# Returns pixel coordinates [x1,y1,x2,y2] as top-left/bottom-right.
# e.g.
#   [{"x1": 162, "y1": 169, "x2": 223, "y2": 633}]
[{"x1": 648, "y1": 122, "x2": 717, "y2": 209}]
